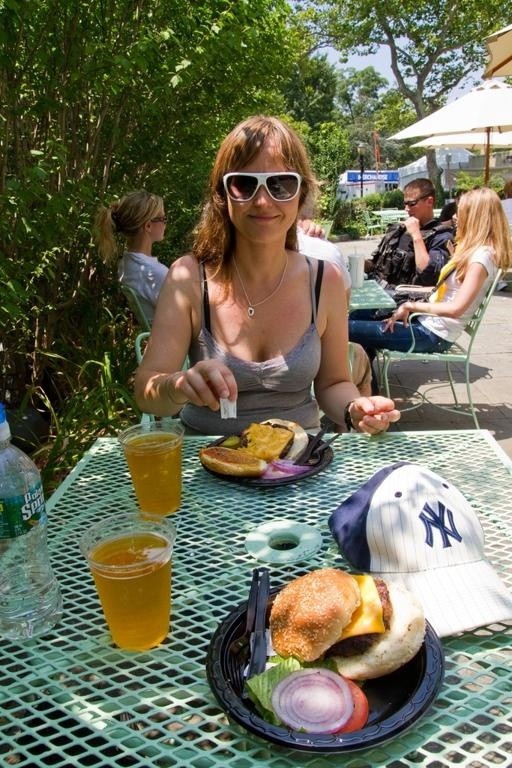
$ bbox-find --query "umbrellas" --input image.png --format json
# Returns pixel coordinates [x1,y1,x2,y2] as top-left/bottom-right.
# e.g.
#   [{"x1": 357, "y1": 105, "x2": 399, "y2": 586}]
[
  {"x1": 410, "y1": 132, "x2": 512, "y2": 149},
  {"x1": 482, "y1": 22, "x2": 512, "y2": 80},
  {"x1": 386, "y1": 77, "x2": 512, "y2": 189}
]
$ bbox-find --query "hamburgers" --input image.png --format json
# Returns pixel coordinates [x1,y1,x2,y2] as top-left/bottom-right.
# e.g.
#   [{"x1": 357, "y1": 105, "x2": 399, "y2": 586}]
[
  {"x1": 269, "y1": 568, "x2": 427, "y2": 682},
  {"x1": 199, "y1": 418, "x2": 309, "y2": 477}
]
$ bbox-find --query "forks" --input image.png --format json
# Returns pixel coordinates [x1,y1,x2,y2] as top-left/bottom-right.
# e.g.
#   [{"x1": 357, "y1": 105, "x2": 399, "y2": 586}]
[
  {"x1": 229, "y1": 567, "x2": 272, "y2": 696},
  {"x1": 292, "y1": 427, "x2": 341, "y2": 465}
]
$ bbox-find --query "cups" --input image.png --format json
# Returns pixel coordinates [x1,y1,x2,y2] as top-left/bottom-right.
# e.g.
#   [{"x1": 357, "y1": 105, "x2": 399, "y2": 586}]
[
  {"x1": 117, "y1": 420, "x2": 187, "y2": 521},
  {"x1": 347, "y1": 254, "x2": 364, "y2": 288},
  {"x1": 78, "y1": 508, "x2": 178, "y2": 653}
]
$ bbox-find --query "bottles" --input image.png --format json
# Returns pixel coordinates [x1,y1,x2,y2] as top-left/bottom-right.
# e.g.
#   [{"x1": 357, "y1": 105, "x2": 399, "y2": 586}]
[{"x1": 0, "y1": 402, "x2": 63, "y2": 647}]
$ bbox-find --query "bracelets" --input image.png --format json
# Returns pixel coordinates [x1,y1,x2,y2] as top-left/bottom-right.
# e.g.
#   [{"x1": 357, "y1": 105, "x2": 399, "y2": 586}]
[
  {"x1": 164, "y1": 371, "x2": 192, "y2": 404},
  {"x1": 345, "y1": 400, "x2": 358, "y2": 431}
]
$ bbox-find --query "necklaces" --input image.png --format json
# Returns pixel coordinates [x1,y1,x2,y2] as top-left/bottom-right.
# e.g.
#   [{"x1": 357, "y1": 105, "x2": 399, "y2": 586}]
[{"x1": 231, "y1": 249, "x2": 288, "y2": 316}]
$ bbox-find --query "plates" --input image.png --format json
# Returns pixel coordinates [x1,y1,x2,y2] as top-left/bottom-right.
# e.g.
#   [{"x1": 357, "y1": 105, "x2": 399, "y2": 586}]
[
  {"x1": 206, "y1": 430, "x2": 336, "y2": 488},
  {"x1": 204, "y1": 567, "x2": 447, "y2": 756}
]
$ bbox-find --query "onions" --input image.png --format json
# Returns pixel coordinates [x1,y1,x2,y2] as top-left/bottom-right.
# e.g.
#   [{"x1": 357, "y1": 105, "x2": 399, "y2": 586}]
[{"x1": 270, "y1": 664, "x2": 352, "y2": 735}]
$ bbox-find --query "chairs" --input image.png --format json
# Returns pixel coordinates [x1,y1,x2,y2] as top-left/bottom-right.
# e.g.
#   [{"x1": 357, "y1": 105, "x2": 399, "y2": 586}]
[
  {"x1": 134, "y1": 333, "x2": 356, "y2": 433},
  {"x1": 375, "y1": 266, "x2": 506, "y2": 428},
  {"x1": 363, "y1": 207, "x2": 401, "y2": 240},
  {"x1": 118, "y1": 283, "x2": 192, "y2": 428}
]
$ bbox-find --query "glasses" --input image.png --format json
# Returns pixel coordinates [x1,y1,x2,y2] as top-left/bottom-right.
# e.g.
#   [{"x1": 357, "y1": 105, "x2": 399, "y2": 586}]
[
  {"x1": 402, "y1": 194, "x2": 430, "y2": 207},
  {"x1": 222, "y1": 171, "x2": 304, "y2": 203}
]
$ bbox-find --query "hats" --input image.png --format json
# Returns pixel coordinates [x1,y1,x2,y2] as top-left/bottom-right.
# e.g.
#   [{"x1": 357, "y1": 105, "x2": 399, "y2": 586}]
[{"x1": 328, "y1": 459, "x2": 512, "y2": 639}]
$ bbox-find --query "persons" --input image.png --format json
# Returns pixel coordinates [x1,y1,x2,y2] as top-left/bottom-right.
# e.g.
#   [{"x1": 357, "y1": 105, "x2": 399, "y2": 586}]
[
  {"x1": 494, "y1": 179, "x2": 512, "y2": 292},
  {"x1": 94, "y1": 187, "x2": 172, "y2": 328},
  {"x1": 438, "y1": 189, "x2": 469, "y2": 218},
  {"x1": 348, "y1": 185, "x2": 512, "y2": 395},
  {"x1": 133, "y1": 112, "x2": 402, "y2": 439},
  {"x1": 295, "y1": 216, "x2": 375, "y2": 399},
  {"x1": 363, "y1": 178, "x2": 454, "y2": 289}
]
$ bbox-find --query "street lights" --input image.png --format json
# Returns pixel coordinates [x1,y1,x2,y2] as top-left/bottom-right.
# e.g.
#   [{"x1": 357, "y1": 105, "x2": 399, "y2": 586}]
[
  {"x1": 357, "y1": 142, "x2": 367, "y2": 198},
  {"x1": 444, "y1": 152, "x2": 454, "y2": 200}
]
